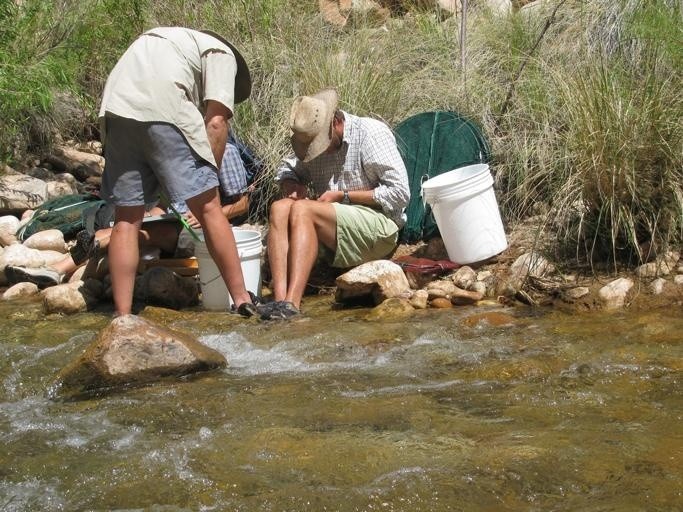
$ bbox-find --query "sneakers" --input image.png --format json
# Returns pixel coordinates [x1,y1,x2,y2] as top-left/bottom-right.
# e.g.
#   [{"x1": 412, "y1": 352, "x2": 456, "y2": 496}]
[
  {"x1": 231, "y1": 291, "x2": 304, "y2": 324},
  {"x1": 69, "y1": 230, "x2": 101, "y2": 267},
  {"x1": 4, "y1": 264, "x2": 66, "y2": 289}
]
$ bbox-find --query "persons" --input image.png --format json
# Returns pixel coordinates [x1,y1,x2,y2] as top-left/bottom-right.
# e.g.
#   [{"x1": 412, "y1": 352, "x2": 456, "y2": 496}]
[
  {"x1": 4, "y1": 140, "x2": 250, "y2": 289},
  {"x1": 238, "y1": 96, "x2": 411, "y2": 321},
  {"x1": 99, "y1": 26, "x2": 265, "y2": 320}
]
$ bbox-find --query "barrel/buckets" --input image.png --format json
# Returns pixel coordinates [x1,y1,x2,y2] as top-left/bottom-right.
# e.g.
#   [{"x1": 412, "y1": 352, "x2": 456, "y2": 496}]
[
  {"x1": 421, "y1": 163, "x2": 508, "y2": 267},
  {"x1": 193, "y1": 230, "x2": 262, "y2": 311}
]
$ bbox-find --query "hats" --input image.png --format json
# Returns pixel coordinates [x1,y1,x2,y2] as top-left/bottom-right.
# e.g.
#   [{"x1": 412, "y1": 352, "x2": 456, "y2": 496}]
[
  {"x1": 289, "y1": 88, "x2": 339, "y2": 163},
  {"x1": 198, "y1": 29, "x2": 252, "y2": 104}
]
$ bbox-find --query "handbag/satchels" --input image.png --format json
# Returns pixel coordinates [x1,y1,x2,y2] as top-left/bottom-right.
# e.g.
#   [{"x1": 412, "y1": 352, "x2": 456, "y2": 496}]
[
  {"x1": 226, "y1": 135, "x2": 262, "y2": 183},
  {"x1": 19, "y1": 194, "x2": 105, "y2": 240}
]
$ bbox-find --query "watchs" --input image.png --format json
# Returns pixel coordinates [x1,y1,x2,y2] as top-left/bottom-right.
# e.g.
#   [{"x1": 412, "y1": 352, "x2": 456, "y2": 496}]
[{"x1": 343, "y1": 190, "x2": 350, "y2": 205}]
